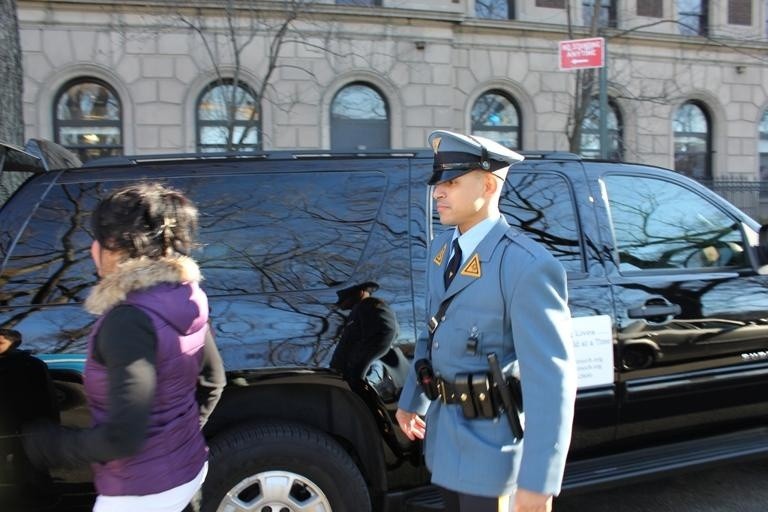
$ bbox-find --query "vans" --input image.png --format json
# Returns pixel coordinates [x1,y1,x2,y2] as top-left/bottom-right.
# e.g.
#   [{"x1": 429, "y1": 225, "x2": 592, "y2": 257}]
[{"x1": 0, "y1": 137, "x2": 768, "y2": 511}]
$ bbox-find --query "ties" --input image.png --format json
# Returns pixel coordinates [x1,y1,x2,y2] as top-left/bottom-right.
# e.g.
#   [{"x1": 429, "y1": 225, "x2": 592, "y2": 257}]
[{"x1": 443, "y1": 238, "x2": 462, "y2": 292}]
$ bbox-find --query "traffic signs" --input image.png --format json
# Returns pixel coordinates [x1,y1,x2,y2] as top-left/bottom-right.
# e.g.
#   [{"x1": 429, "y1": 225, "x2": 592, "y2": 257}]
[{"x1": 557, "y1": 37, "x2": 605, "y2": 70}]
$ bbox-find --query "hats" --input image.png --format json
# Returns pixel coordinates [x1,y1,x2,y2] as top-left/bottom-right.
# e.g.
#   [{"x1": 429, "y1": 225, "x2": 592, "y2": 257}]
[
  {"x1": 335, "y1": 282, "x2": 379, "y2": 305},
  {"x1": 427, "y1": 130, "x2": 525, "y2": 185}
]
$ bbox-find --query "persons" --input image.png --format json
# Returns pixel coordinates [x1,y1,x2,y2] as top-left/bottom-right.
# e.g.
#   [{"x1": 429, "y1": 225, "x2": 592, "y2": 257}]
[
  {"x1": 395, "y1": 129, "x2": 577, "y2": 512},
  {"x1": 0, "y1": 329, "x2": 63, "y2": 512},
  {"x1": 331, "y1": 281, "x2": 405, "y2": 470},
  {"x1": 77, "y1": 179, "x2": 227, "y2": 512}
]
